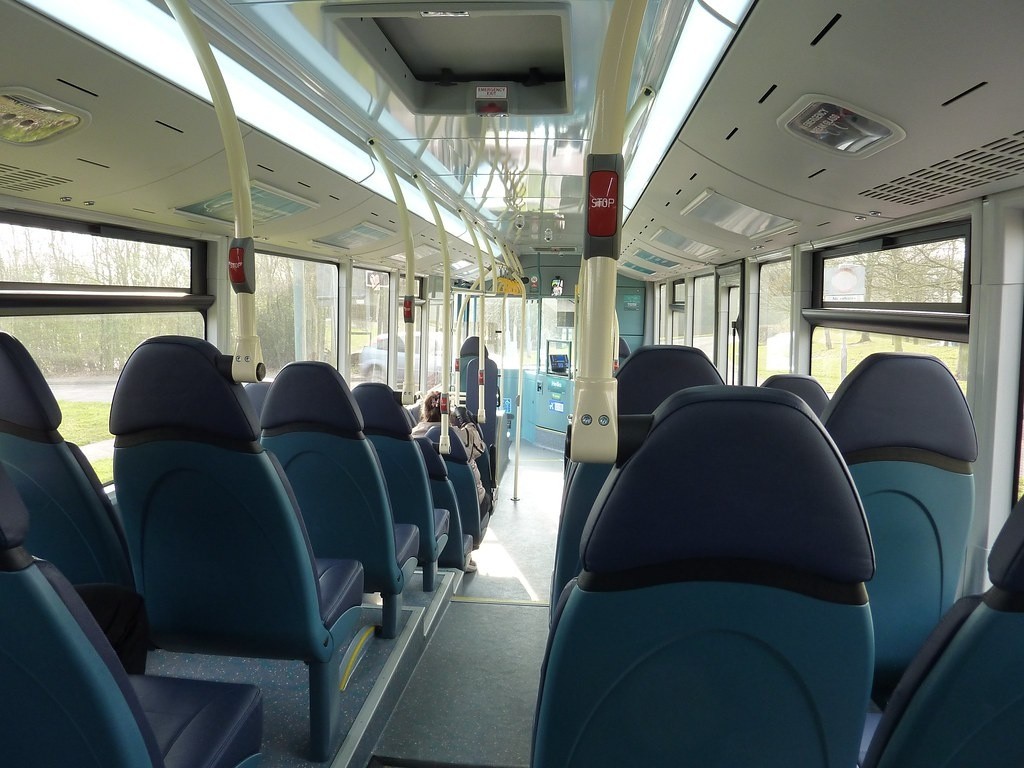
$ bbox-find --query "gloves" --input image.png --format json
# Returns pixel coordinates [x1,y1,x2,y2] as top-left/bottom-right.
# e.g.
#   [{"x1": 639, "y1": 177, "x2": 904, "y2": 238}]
[{"x1": 454, "y1": 407, "x2": 468, "y2": 426}]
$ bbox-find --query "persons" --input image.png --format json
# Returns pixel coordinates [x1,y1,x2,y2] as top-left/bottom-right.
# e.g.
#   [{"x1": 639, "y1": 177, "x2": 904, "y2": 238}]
[{"x1": 411, "y1": 390, "x2": 491, "y2": 573}]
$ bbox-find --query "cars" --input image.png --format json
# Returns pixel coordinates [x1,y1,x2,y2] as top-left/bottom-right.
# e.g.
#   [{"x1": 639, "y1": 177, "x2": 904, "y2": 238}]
[{"x1": 357, "y1": 329, "x2": 462, "y2": 382}]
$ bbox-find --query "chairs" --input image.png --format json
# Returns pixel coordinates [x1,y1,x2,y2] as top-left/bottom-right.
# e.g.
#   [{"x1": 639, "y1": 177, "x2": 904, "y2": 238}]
[
  {"x1": 0, "y1": 336, "x2": 489, "y2": 768},
  {"x1": 531, "y1": 338, "x2": 1024, "y2": 768}
]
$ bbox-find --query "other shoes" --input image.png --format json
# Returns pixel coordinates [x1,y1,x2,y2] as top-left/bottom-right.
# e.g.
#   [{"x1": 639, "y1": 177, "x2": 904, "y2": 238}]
[{"x1": 466, "y1": 559, "x2": 477, "y2": 572}]
